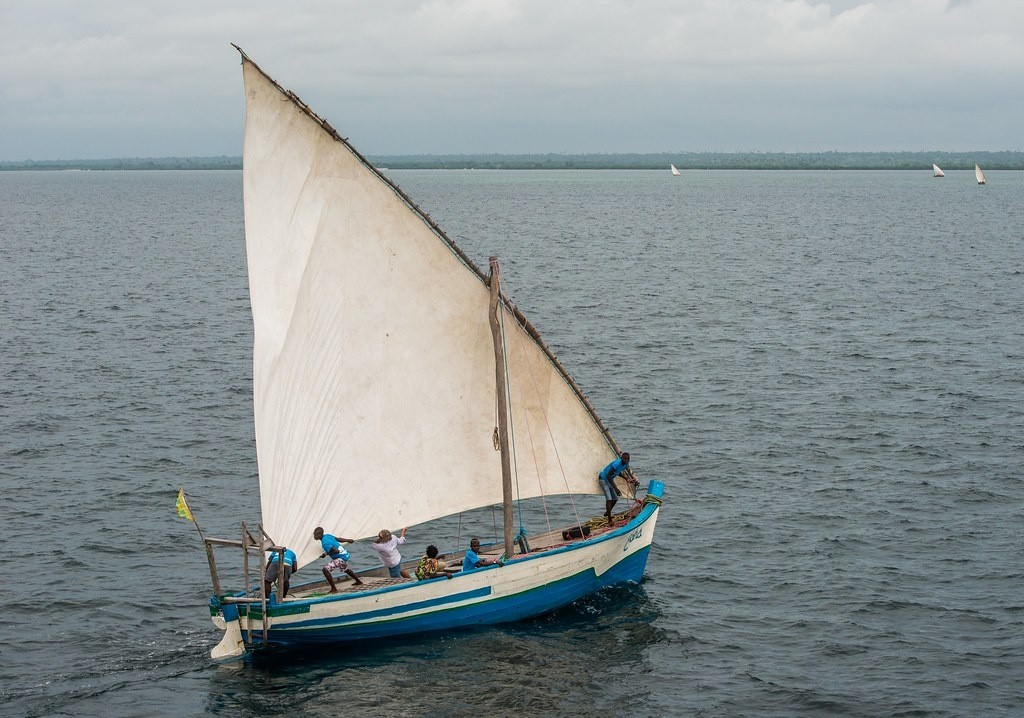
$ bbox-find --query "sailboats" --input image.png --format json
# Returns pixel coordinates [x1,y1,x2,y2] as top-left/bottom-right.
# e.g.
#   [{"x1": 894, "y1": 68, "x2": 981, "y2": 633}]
[
  {"x1": 933, "y1": 163, "x2": 946, "y2": 177},
  {"x1": 671, "y1": 164, "x2": 681, "y2": 176},
  {"x1": 176, "y1": 43, "x2": 665, "y2": 669},
  {"x1": 974, "y1": 163, "x2": 987, "y2": 185}
]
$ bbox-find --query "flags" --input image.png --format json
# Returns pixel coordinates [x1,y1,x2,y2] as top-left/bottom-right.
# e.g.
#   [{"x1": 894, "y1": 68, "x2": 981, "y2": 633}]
[{"x1": 175, "y1": 487, "x2": 194, "y2": 520}]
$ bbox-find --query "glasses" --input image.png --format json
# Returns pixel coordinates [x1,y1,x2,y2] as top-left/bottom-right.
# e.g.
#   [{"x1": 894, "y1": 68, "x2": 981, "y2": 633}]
[{"x1": 379, "y1": 533, "x2": 382, "y2": 539}]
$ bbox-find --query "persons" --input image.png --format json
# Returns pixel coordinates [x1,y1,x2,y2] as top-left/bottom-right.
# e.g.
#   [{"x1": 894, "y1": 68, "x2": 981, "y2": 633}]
[
  {"x1": 313, "y1": 527, "x2": 363, "y2": 594},
  {"x1": 463, "y1": 538, "x2": 505, "y2": 571},
  {"x1": 264, "y1": 549, "x2": 297, "y2": 599},
  {"x1": 415, "y1": 544, "x2": 453, "y2": 580},
  {"x1": 371, "y1": 527, "x2": 412, "y2": 578},
  {"x1": 599, "y1": 452, "x2": 640, "y2": 527}
]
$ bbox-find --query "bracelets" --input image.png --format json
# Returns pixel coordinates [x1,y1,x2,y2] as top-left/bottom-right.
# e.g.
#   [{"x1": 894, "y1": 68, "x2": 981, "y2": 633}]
[{"x1": 490, "y1": 558, "x2": 496, "y2": 564}]
